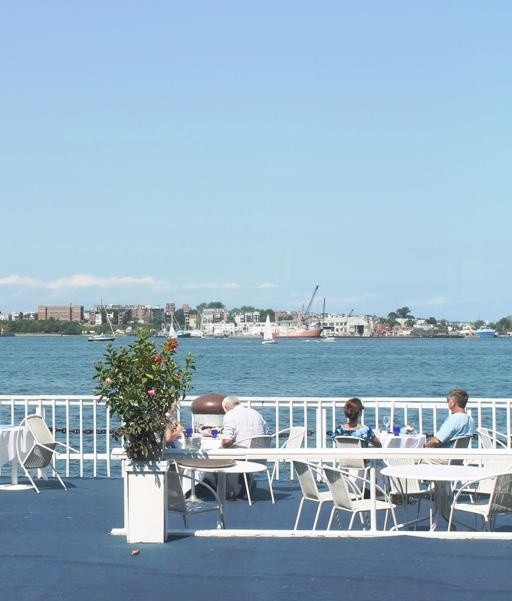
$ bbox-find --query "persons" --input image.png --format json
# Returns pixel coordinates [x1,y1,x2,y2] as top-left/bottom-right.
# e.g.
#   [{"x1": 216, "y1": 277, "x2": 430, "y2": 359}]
[
  {"x1": 220, "y1": 396, "x2": 268, "y2": 502},
  {"x1": 418, "y1": 389, "x2": 474, "y2": 488},
  {"x1": 161, "y1": 398, "x2": 205, "y2": 498},
  {"x1": 330, "y1": 397, "x2": 418, "y2": 506}
]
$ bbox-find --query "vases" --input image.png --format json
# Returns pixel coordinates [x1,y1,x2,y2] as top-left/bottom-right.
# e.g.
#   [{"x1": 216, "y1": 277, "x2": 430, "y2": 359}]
[{"x1": 120, "y1": 459, "x2": 172, "y2": 545}]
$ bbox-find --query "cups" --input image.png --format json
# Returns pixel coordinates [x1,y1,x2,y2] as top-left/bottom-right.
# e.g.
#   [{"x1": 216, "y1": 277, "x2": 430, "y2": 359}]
[
  {"x1": 393, "y1": 427, "x2": 400, "y2": 436},
  {"x1": 209, "y1": 430, "x2": 218, "y2": 438},
  {"x1": 186, "y1": 428, "x2": 192, "y2": 437}
]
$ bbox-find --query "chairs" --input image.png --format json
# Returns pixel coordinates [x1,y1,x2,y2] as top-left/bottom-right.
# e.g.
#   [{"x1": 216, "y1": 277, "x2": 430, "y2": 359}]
[
  {"x1": 170, "y1": 427, "x2": 512, "y2": 505},
  {"x1": 323, "y1": 465, "x2": 400, "y2": 533},
  {"x1": 447, "y1": 472, "x2": 512, "y2": 533},
  {"x1": 169, "y1": 458, "x2": 225, "y2": 531},
  {"x1": 16, "y1": 414, "x2": 81, "y2": 494},
  {"x1": 453, "y1": 458, "x2": 512, "y2": 504},
  {"x1": 382, "y1": 458, "x2": 436, "y2": 533},
  {"x1": 292, "y1": 459, "x2": 364, "y2": 532}
]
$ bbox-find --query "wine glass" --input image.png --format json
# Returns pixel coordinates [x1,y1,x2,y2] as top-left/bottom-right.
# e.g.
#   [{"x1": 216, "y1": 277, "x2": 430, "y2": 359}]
[
  {"x1": 383, "y1": 416, "x2": 390, "y2": 434},
  {"x1": 193, "y1": 415, "x2": 200, "y2": 437}
]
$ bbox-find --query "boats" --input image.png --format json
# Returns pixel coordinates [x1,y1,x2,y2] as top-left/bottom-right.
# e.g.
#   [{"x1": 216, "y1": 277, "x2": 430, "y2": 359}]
[
  {"x1": 326, "y1": 335, "x2": 336, "y2": 342},
  {"x1": 87, "y1": 331, "x2": 117, "y2": 343},
  {"x1": 472, "y1": 326, "x2": 496, "y2": 338},
  {"x1": 260, "y1": 319, "x2": 324, "y2": 337}
]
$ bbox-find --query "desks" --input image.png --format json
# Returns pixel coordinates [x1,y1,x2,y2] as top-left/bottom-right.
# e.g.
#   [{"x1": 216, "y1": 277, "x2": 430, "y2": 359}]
[
  {"x1": 380, "y1": 463, "x2": 487, "y2": 532},
  {"x1": 178, "y1": 457, "x2": 268, "y2": 529}
]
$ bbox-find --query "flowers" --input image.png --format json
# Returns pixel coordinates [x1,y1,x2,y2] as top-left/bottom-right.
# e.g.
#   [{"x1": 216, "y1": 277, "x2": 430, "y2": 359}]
[{"x1": 92, "y1": 328, "x2": 199, "y2": 474}]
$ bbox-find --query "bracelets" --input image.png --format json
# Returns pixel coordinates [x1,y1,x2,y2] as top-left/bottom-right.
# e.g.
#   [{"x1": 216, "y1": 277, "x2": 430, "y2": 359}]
[{"x1": 422, "y1": 444, "x2": 425, "y2": 449}]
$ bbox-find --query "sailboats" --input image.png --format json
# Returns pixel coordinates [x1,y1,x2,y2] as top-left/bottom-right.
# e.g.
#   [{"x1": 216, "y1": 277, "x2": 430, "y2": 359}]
[
  {"x1": 261, "y1": 313, "x2": 278, "y2": 345},
  {"x1": 156, "y1": 315, "x2": 190, "y2": 338}
]
